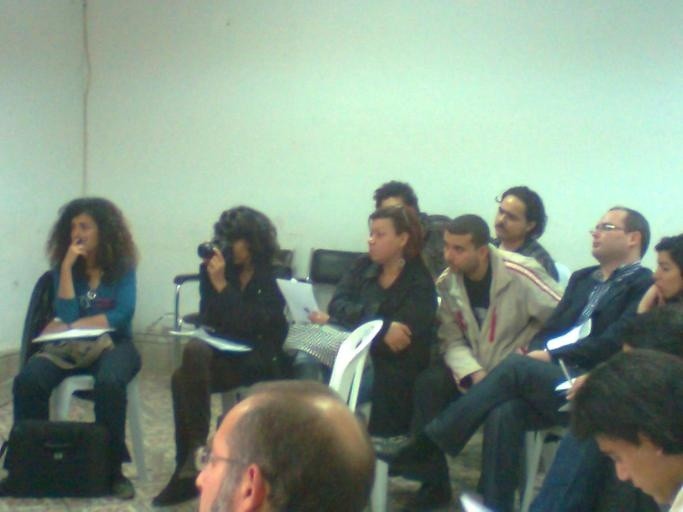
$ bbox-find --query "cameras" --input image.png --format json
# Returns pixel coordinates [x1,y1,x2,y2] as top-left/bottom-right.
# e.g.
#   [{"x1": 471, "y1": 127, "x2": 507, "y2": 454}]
[{"x1": 198, "y1": 239, "x2": 229, "y2": 260}]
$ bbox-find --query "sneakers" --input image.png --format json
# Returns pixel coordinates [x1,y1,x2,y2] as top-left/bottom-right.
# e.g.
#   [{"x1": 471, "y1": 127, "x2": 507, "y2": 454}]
[
  {"x1": 401, "y1": 483, "x2": 451, "y2": 511},
  {"x1": 113, "y1": 477, "x2": 135, "y2": 500},
  {"x1": 152, "y1": 473, "x2": 200, "y2": 507}
]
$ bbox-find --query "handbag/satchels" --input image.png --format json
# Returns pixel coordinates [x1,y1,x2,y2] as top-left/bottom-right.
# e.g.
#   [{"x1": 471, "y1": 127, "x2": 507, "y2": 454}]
[
  {"x1": 0, "y1": 421, "x2": 112, "y2": 497},
  {"x1": 39, "y1": 334, "x2": 115, "y2": 366},
  {"x1": 283, "y1": 322, "x2": 373, "y2": 370}
]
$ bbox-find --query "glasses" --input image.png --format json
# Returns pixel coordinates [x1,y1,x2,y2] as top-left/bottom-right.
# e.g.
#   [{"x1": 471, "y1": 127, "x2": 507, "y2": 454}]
[{"x1": 596, "y1": 223, "x2": 631, "y2": 233}]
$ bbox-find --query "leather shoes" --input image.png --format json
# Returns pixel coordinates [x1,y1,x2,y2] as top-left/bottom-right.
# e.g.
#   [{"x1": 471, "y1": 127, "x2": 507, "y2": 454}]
[{"x1": 370, "y1": 430, "x2": 438, "y2": 474}]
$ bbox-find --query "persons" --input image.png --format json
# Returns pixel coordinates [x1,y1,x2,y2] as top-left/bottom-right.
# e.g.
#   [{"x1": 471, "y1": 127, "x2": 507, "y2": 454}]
[
  {"x1": 387, "y1": 214, "x2": 570, "y2": 511},
  {"x1": 2, "y1": 198, "x2": 138, "y2": 498},
  {"x1": 191, "y1": 380, "x2": 374, "y2": 512},
  {"x1": 303, "y1": 207, "x2": 439, "y2": 441},
  {"x1": 371, "y1": 202, "x2": 659, "y2": 510},
  {"x1": 524, "y1": 231, "x2": 683, "y2": 512},
  {"x1": 151, "y1": 207, "x2": 289, "y2": 505},
  {"x1": 368, "y1": 180, "x2": 469, "y2": 281},
  {"x1": 485, "y1": 182, "x2": 563, "y2": 284}
]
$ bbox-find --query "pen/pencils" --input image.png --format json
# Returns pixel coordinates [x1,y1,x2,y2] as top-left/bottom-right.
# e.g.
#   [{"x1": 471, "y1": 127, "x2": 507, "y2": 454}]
[{"x1": 559, "y1": 358, "x2": 572, "y2": 386}]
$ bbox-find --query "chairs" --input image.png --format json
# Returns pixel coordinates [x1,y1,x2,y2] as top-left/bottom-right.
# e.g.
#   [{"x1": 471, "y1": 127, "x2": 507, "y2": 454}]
[
  {"x1": 173, "y1": 250, "x2": 293, "y2": 346},
  {"x1": 329, "y1": 319, "x2": 384, "y2": 412},
  {"x1": 517, "y1": 425, "x2": 568, "y2": 511},
  {"x1": 52, "y1": 375, "x2": 145, "y2": 478},
  {"x1": 301, "y1": 247, "x2": 368, "y2": 328}
]
